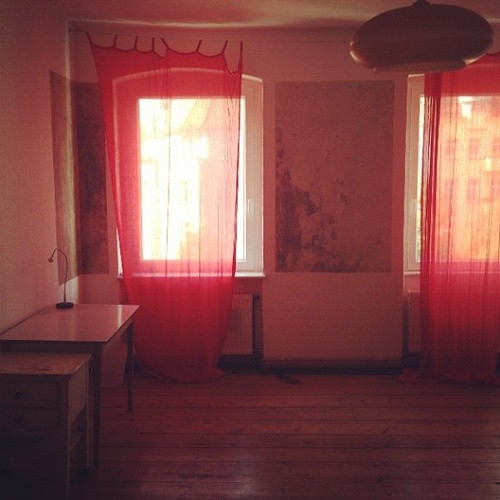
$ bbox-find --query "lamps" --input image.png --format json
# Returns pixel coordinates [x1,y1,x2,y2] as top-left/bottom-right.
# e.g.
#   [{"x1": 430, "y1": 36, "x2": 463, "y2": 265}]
[
  {"x1": 47, "y1": 247, "x2": 74, "y2": 309},
  {"x1": 348, "y1": 1, "x2": 495, "y2": 75}
]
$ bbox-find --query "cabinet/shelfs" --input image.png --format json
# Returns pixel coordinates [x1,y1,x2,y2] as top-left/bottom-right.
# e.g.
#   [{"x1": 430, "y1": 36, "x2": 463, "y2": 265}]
[{"x1": 1, "y1": 349, "x2": 90, "y2": 500}]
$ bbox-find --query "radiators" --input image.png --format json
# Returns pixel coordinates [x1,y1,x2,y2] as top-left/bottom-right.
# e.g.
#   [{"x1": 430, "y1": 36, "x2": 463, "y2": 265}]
[
  {"x1": 217, "y1": 293, "x2": 261, "y2": 358},
  {"x1": 405, "y1": 289, "x2": 422, "y2": 355}
]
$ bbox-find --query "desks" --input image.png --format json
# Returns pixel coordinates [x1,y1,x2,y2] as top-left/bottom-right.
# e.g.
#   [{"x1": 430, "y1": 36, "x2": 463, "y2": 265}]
[{"x1": 1, "y1": 302, "x2": 141, "y2": 470}]
